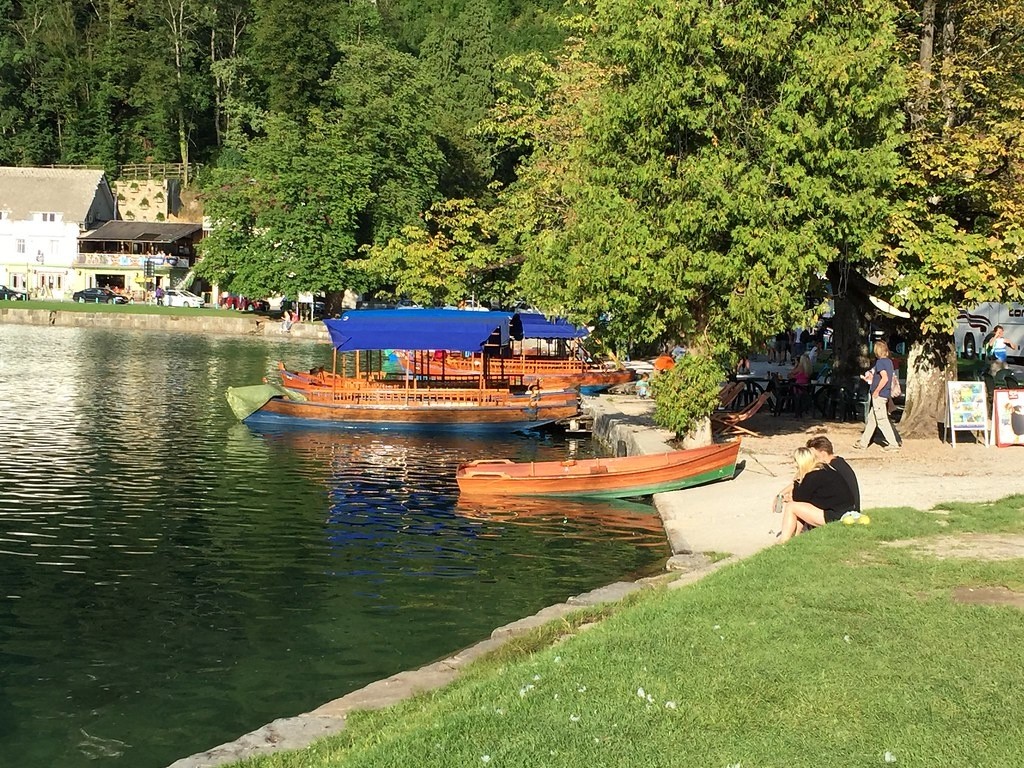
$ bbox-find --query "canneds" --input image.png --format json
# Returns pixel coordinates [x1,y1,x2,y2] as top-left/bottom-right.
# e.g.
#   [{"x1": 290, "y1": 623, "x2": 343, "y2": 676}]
[{"x1": 775, "y1": 494, "x2": 784, "y2": 513}]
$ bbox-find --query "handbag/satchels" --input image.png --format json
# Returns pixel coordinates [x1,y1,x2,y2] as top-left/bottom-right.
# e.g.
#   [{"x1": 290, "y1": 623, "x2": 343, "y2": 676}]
[
  {"x1": 890, "y1": 371, "x2": 901, "y2": 399},
  {"x1": 817, "y1": 364, "x2": 833, "y2": 384}
]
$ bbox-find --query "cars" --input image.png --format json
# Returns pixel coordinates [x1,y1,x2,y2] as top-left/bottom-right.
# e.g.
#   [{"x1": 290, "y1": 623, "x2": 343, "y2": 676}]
[
  {"x1": 73, "y1": 288, "x2": 129, "y2": 305},
  {"x1": 0, "y1": 285, "x2": 30, "y2": 301},
  {"x1": 159, "y1": 290, "x2": 205, "y2": 307}
]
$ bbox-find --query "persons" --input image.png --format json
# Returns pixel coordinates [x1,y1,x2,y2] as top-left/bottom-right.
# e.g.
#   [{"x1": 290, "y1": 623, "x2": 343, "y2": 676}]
[
  {"x1": 635, "y1": 372, "x2": 651, "y2": 399},
  {"x1": 147, "y1": 250, "x2": 152, "y2": 255},
  {"x1": 772, "y1": 436, "x2": 860, "y2": 544},
  {"x1": 148, "y1": 285, "x2": 165, "y2": 305},
  {"x1": 788, "y1": 354, "x2": 813, "y2": 396},
  {"x1": 281, "y1": 310, "x2": 298, "y2": 330},
  {"x1": 766, "y1": 324, "x2": 794, "y2": 367},
  {"x1": 157, "y1": 250, "x2": 165, "y2": 256},
  {"x1": 853, "y1": 340, "x2": 903, "y2": 449},
  {"x1": 989, "y1": 324, "x2": 1016, "y2": 360}
]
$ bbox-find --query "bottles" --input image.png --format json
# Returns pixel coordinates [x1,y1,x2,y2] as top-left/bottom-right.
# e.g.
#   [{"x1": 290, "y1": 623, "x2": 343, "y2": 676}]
[
  {"x1": 865, "y1": 370, "x2": 874, "y2": 385},
  {"x1": 776, "y1": 491, "x2": 784, "y2": 513}
]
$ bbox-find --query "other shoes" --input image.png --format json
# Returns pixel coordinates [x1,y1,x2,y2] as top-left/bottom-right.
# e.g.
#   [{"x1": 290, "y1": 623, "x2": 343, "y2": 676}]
[
  {"x1": 768, "y1": 359, "x2": 796, "y2": 366},
  {"x1": 853, "y1": 442, "x2": 866, "y2": 451},
  {"x1": 881, "y1": 444, "x2": 901, "y2": 453}
]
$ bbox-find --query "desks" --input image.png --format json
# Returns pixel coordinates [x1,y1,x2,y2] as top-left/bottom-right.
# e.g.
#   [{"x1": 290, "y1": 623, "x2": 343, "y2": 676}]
[{"x1": 727, "y1": 375, "x2": 776, "y2": 411}]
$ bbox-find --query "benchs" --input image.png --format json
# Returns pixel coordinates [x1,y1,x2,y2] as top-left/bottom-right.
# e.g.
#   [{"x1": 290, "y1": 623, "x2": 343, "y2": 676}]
[{"x1": 590, "y1": 465, "x2": 608, "y2": 475}]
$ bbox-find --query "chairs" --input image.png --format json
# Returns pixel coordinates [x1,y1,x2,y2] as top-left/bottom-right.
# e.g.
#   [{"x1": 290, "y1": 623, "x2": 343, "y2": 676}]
[{"x1": 774, "y1": 378, "x2": 905, "y2": 423}]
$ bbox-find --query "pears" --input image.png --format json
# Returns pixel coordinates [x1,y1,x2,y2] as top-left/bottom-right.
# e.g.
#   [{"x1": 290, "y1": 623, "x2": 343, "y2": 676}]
[
  {"x1": 858, "y1": 513, "x2": 870, "y2": 525},
  {"x1": 843, "y1": 514, "x2": 854, "y2": 524}
]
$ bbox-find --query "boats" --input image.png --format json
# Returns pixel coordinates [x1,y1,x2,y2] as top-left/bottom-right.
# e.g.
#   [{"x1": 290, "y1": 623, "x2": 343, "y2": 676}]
[
  {"x1": 399, "y1": 311, "x2": 637, "y2": 395},
  {"x1": 225, "y1": 309, "x2": 582, "y2": 434},
  {"x1": 456, "y1": 435, "x2": 742, "y2": 499}
]
